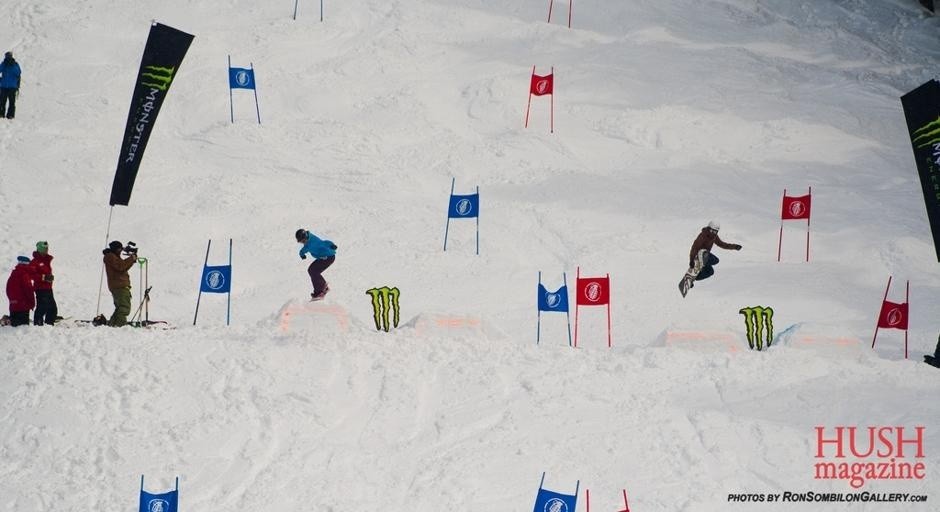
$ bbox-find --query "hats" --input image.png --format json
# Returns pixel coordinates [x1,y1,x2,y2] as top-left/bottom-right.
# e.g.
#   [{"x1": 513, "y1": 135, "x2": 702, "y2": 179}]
[
  {"x1": 17, "y1": 255, "x2": 31, "y2": 264},
  {"x1": 108, "y1": 241, "x2": 123, "y2": 250},
  {"x1": 36, "y1": 241, "x2": 49, "y2": 253}
]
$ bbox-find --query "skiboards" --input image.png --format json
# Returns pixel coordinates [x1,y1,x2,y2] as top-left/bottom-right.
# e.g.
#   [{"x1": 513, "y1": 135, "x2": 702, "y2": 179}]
[{"x1": 74, "y1": 320, "x2": 176, "y2": 330}]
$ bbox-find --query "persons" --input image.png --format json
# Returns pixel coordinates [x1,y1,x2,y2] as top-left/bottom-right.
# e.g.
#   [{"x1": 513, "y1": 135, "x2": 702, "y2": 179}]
[
  {"x1": 102, "y1": 241, "x2": 137, "y2": 326},
  {"x1": 0, "y1": 51, "x2": 21, "y2": 119},
  {"x1": 690, "y1": 219, "x2": 742, "y2": 288},
  {"x1": 6, "y1": 256, "x2": 36, "y2": 327},
  {"x1": 29, "y1": 241, "x2": 58, "y2": 327},
  {"x1": 296, "y1": 228, "x2": 337, "y2": 297}
]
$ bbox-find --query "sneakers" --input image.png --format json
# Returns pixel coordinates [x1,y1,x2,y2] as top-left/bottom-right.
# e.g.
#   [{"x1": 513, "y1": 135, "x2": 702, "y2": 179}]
[{"x1": 311, "y1": 287, "x2": 329, "y2": 298}]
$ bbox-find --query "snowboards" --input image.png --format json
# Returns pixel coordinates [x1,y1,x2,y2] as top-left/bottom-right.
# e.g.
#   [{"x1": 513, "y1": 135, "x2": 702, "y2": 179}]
[
  {"x1": 679, "y1": 249, "x2": 709, "y2": 298},
  {"x1": 311, "y1": 286, "x2": 329, "y2": 300}
]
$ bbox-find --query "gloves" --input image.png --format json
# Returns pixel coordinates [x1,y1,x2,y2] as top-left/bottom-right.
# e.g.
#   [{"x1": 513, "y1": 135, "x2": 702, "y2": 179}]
[{"x1": 42, "y1": 274, "x2": 55, "y2": 282}]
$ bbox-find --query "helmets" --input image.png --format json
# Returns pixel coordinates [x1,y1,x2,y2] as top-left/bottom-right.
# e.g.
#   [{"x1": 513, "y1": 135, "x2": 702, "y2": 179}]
[
  {"x1": 295, "y1": 228, "x2": 309, "y2": 243},
  {"x1": 708, "y1": 219, "x2": 720, "y2": 230}
]
